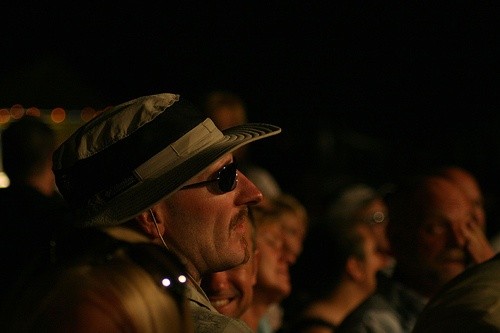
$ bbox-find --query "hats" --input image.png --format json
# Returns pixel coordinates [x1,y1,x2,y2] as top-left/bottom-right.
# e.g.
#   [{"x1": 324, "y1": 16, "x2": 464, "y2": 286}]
[{"x1": 47, "y1": 92, "x2": 283, "y2": 228}]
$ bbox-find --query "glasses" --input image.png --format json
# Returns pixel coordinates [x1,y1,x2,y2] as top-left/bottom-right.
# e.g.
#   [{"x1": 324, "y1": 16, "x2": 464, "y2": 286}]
[{"x1": 178, "y1": 156, "x2": 247, "y2": 195}]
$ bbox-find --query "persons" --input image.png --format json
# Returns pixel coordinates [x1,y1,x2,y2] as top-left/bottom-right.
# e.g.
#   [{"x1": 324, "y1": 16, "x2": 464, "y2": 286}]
[
  {"x1": 412, "y1": 251, "x2": 500, "y2": 333},
  {"x1": 0, "y1": 115, "x2": 69, "y2": 292},
  {"x1": 334, "y1": 176, "x2": 500, "y2": 333},
  {"x1": 10, "y1": 93, "x2": 282, "y2": 333},
  {"x1": 198, "y1": 93, "x2": 397, "y2": 333},
  {"x1": 429, "y1": 165, "x2": 500, "y2": 253}
]
